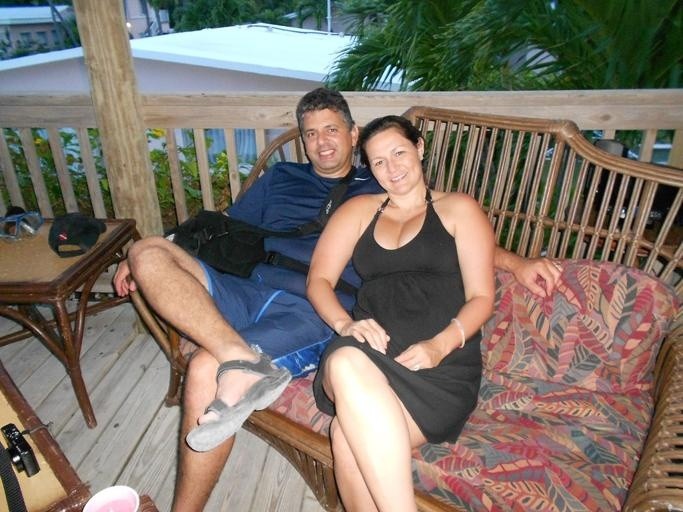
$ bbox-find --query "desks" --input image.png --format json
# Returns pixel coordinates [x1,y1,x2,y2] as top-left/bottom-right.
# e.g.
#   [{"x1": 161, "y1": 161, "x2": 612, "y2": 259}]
[{"x1": 0, "y1": 214, "x2": 142, "y2": 431}]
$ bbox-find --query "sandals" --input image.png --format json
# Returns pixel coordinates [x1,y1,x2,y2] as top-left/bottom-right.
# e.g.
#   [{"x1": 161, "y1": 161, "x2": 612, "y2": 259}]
[{"x1": 186, "y1": 349, "x2": 292, "y2": 452}]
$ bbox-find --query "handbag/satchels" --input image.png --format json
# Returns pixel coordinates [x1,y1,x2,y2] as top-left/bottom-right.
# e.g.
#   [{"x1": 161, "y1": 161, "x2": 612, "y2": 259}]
[{"x1": 171, "y1": 210, "x2": 265, "y2": 278}]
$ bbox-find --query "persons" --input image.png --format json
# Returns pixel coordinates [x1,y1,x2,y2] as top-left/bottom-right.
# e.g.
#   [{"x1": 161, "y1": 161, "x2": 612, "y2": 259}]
[
  {"x1": 305, "y1": 116, "x2": 495, "y2": 512},
  {"x1": 112, "y1": 87, "x2": 565, "y2": 512}
]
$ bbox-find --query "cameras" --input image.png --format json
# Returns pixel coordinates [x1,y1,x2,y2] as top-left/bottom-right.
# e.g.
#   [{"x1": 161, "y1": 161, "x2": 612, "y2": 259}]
[{"x1": 0, "y1": 422, "x2": 41, "y2": 478}]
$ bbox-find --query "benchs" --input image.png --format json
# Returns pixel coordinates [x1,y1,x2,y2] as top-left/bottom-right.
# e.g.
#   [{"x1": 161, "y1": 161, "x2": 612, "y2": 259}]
[{"x1": 126, "y1": 102, "x2": 682, "y2": 512}]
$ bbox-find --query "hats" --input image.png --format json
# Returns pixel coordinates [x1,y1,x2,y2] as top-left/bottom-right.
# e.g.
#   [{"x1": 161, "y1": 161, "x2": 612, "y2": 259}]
[{"x1": 50, "y1": 213, "x2": 106, "y2": 258}]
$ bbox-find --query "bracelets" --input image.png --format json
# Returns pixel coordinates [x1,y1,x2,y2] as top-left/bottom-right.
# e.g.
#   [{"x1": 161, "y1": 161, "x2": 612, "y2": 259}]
[{"x1": 451, "y1": 318, "x2": 465, "y2": 348}]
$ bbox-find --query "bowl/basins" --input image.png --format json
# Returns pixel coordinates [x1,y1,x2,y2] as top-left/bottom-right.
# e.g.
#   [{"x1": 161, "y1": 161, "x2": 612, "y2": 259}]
[{"x1": 82, "y1": 485, "x2": 139, "y2": 512}]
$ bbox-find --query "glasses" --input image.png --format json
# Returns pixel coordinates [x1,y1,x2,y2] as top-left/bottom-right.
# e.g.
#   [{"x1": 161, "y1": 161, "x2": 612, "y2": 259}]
[{"x1": 0, "y1": 209, "x2": 44, "y2": 242}]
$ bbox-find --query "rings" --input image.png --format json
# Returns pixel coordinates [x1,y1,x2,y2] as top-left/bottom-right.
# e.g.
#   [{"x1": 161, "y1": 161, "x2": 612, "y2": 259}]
[
  {"x1": 414, "y1": 364, "x2": 420, "y2": 371},
  {"x1": 556, "y1": 263, "x2": 562, "y2": 267}
]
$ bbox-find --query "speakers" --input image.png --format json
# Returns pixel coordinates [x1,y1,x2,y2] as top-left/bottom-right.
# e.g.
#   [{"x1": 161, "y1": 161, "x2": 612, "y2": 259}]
[{"x1": 583, "y1": 139, "x2": 628, "y2": 207}]
